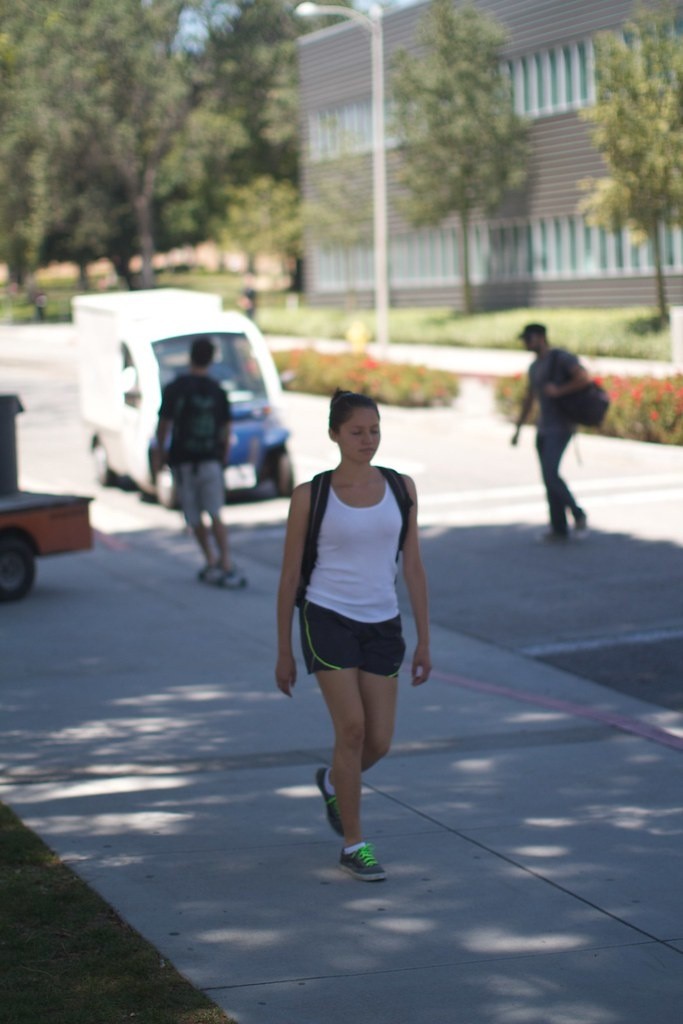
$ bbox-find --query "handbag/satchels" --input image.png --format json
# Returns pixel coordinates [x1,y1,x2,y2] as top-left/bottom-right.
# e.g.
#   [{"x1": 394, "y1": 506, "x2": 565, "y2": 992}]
[{"x1": 547, "y1": 349, "x2": 608, "y2": 423}]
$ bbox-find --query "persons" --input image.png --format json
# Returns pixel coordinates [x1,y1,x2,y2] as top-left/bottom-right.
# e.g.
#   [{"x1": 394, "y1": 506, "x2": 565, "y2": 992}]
[
  {"x1": 151, "y1": 337, "x2": 235, "y2": 573},
  {"x1": 511, "y1": 323, "x2": 594, "y2": 542},
  {"x1": 274, "y1": 389, "x2": 433, "y2": 883}
]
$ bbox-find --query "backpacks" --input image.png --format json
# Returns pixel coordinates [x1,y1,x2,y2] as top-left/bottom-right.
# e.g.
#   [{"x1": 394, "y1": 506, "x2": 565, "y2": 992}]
[{"x1": 170, "y1": 377, "x2": 222, "y2": 459}]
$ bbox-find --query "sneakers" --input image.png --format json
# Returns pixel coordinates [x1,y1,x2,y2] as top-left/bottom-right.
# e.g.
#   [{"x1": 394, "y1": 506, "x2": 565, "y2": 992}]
[
  {"x1": 339, "y1": 842, "x2": 388, "y2": 881},
  {"x1": 315, "y1": 765, "x2": 343, "y2": 836}
]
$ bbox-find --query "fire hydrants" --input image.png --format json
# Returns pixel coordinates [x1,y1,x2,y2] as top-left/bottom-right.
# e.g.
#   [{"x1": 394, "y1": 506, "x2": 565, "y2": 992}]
[{"x1": 346, "y1": 320, "x2": 373, "y2": 358}]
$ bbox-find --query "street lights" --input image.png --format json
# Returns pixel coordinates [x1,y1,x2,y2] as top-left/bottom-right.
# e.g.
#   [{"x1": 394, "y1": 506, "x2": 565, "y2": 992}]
[{"x1": 295, "y1": 2, "x2": 391, "y2": 352}]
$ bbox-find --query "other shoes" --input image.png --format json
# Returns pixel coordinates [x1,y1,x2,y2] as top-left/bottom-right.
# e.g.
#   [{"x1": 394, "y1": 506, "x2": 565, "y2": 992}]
[
  {"x1": 576, "y1": 516, "x2": 589, "y2": 539},
  {"x1": 200, "y1": 564, "x2": 247, "y2": 588},
  {"x1": 538, "y1": 532, "x2": 566, "y2": 544}
]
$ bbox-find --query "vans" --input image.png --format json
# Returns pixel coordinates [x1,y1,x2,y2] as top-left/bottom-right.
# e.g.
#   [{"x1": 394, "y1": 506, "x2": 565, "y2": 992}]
[{"x1": 73, "y1": 288, "x2": 295, "y2": 514}]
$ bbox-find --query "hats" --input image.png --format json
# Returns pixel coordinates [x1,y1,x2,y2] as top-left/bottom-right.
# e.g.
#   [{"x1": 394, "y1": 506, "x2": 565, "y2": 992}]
[{"x1": 518, "y1": 324, "x2": 545, "y2": 338}]
